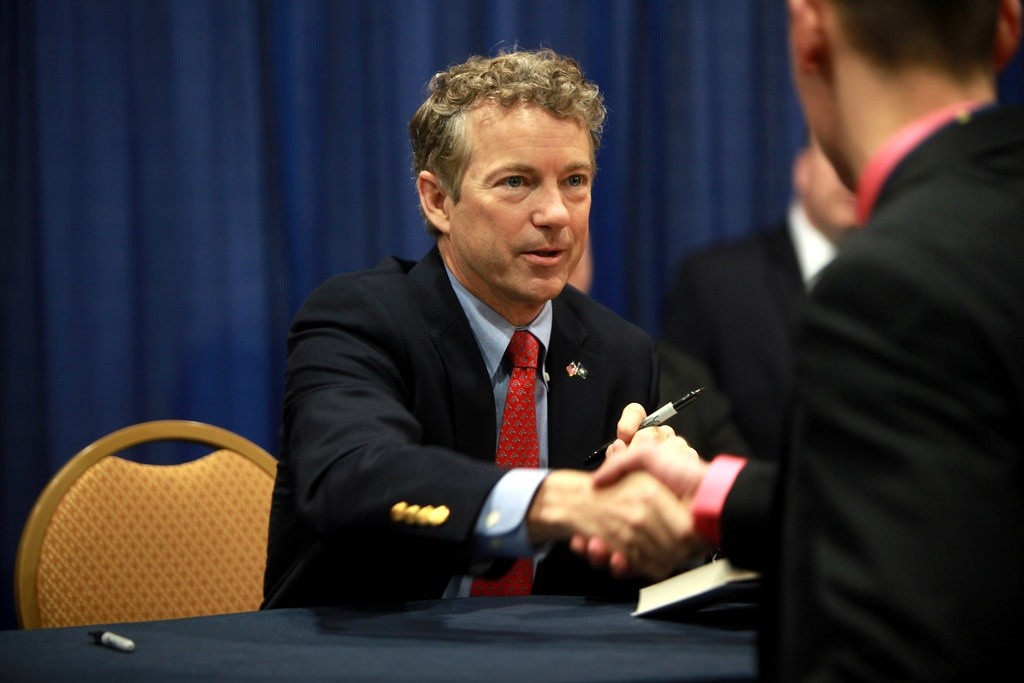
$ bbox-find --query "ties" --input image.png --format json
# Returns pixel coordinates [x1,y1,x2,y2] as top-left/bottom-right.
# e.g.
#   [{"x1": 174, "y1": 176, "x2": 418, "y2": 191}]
[{"x1": 469, "y1": 331, "x2": 541, "y2": 597}]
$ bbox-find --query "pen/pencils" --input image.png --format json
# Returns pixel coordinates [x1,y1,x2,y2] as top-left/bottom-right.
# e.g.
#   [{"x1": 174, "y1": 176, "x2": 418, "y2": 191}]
[
  {"x1": 585, "y1": 387, "x2": 706, "y2": 464},
  {"x1": 86, "y1": 628, "x2": 136, "y2": 652}
]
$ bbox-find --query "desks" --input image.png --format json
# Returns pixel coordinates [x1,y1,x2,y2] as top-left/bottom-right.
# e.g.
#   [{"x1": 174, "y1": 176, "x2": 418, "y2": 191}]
[{"x1": 0, "y1": 595, "x2": 758, "y2": 683}]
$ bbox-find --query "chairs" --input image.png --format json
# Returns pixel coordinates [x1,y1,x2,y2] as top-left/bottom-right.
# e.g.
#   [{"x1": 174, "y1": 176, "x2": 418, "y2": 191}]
[{"x1": 17, "y1": 419, "x2": 281, "y2": 631}]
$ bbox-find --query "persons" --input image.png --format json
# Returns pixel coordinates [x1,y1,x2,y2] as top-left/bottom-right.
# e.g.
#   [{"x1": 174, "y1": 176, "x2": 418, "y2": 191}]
[
  {"x1": 259, "y1": 48, "x2": 854, "y2": 610},
  {"x1": 570, "y1": 0, "x2": 1024, "y2": 683}
]
